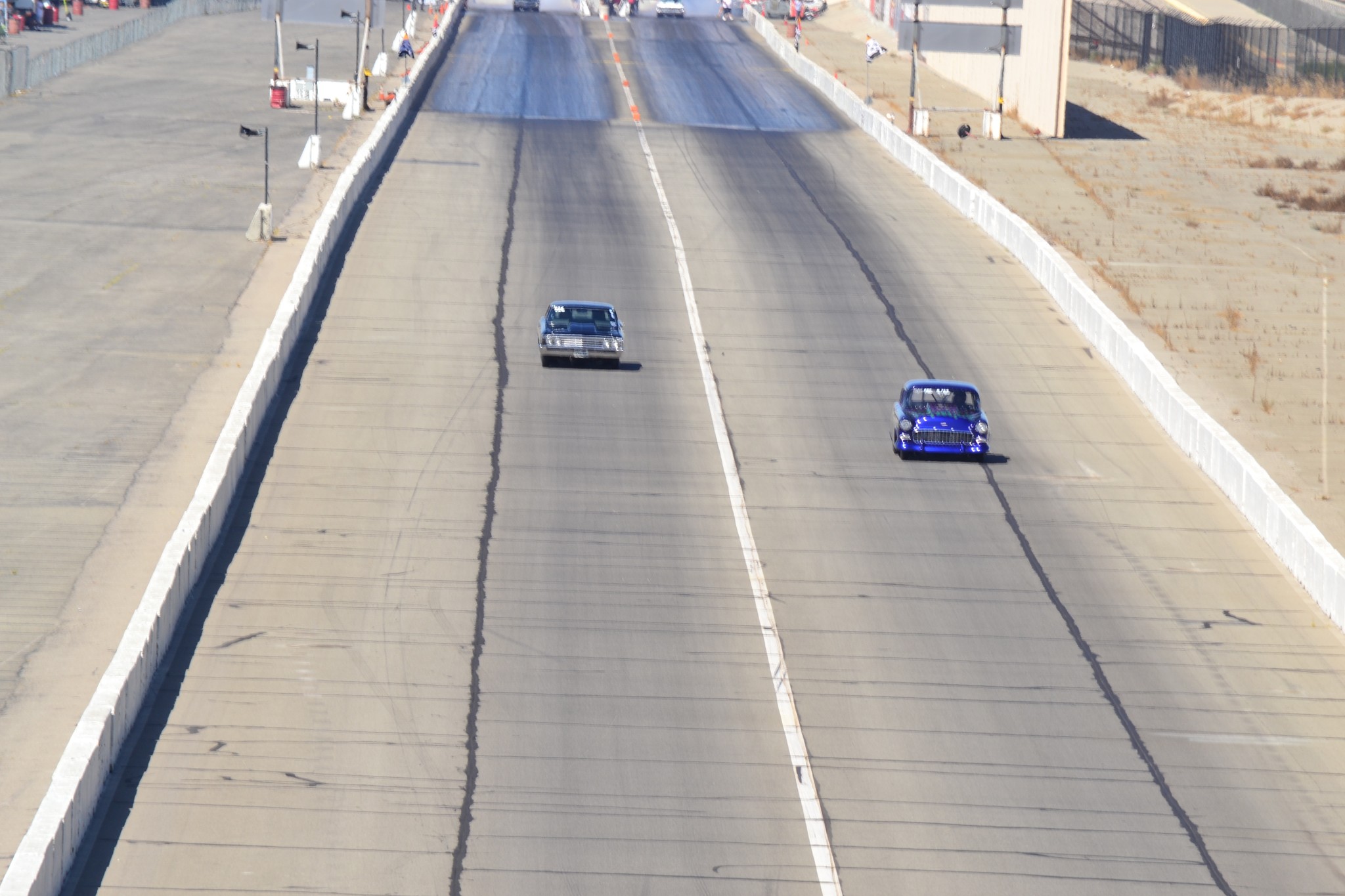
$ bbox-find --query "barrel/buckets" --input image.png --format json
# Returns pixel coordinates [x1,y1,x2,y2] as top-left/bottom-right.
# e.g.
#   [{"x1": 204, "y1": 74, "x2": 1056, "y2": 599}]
[
  {"x1": 13, "y1": 15, "x2": 24, "y2": 31},
  {"x1": 108, "y1": 0, "x2": 118, "y2": 9},
  {"x1": 8, "y1": 18, "x2": 20, "y2": 34},
  {"x1": 46, "y1": 6, "x2": 59, "y2": 23},
  {"x1": 140, "y1": 0, "x2": 150, "y2": 8},
  {"x1": 72, "y1": 0, "x2": 83, "y2": 14}
]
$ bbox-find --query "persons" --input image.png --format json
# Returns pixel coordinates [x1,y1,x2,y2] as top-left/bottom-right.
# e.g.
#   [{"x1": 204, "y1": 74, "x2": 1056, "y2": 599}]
[
  {"x1": 716, "y1": 0, "x2": 734, "y2": 21},
  {"x1": 794, "y1": 0, "x2": 802, "y2": 22},
  {"x1": 950, "y1": 391, "x2": 967, "y2": 408},
  {"x1": 627, "y1": 0, "x2": 644, "y2": 15}
]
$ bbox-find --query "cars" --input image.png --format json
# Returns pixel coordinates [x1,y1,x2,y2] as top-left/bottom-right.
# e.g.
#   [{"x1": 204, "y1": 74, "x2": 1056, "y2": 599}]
[
  {"x1": 887, "y1": 379, "x2": 992, "y2": 460},
  {"x1": 513, "y1": 0, "x2": 540, "y2": 12},
  {"x1": 537, "y1": 299, "x2": 625, "y2": 365},
  {"x1": 656, "y1": 0, "x2": 685, "y2": 18}
]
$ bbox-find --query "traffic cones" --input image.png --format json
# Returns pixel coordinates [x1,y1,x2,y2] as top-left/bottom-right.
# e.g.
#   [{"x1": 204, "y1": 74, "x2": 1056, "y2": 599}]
[
  {"x1": 379, "y1": 83, "x2": 385, "y2": 100},
  {"x1": 782, "y1": 15, "x2": 789, "y2": 26},
  {"x1": 428, "y1": 2, "x2": 434, "y2": 15},
  {"x1": 438, "y1": 0, "x2": 449, "y2": 15},
  {"x1": 762, "y1": 5, "x2": 767, "y2": 18},
  {"x1": 833, "y1": 70, "x2": 838, "y2": 80},
  {"x1": 805, "y1": 37, "x2": 811, "y2": 46},
  {"x1": 433, "y1": 13, "x2": 440, "y2": 28},
  {"x1": 843, "y1": 79, "x2": 848, "y2": 88}
]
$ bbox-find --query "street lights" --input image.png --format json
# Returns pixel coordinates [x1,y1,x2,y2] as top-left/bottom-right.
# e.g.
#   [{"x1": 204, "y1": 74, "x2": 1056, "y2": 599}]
[
  {"x1": 294, "y1": 42, "x2": 318, "y2": 135},
  {"x1": 339, "y1": 9, "x2": 361, "y2": 82},
  {"x1": 236, "y1": 124, "x2": 271, "y2": 209}
]
